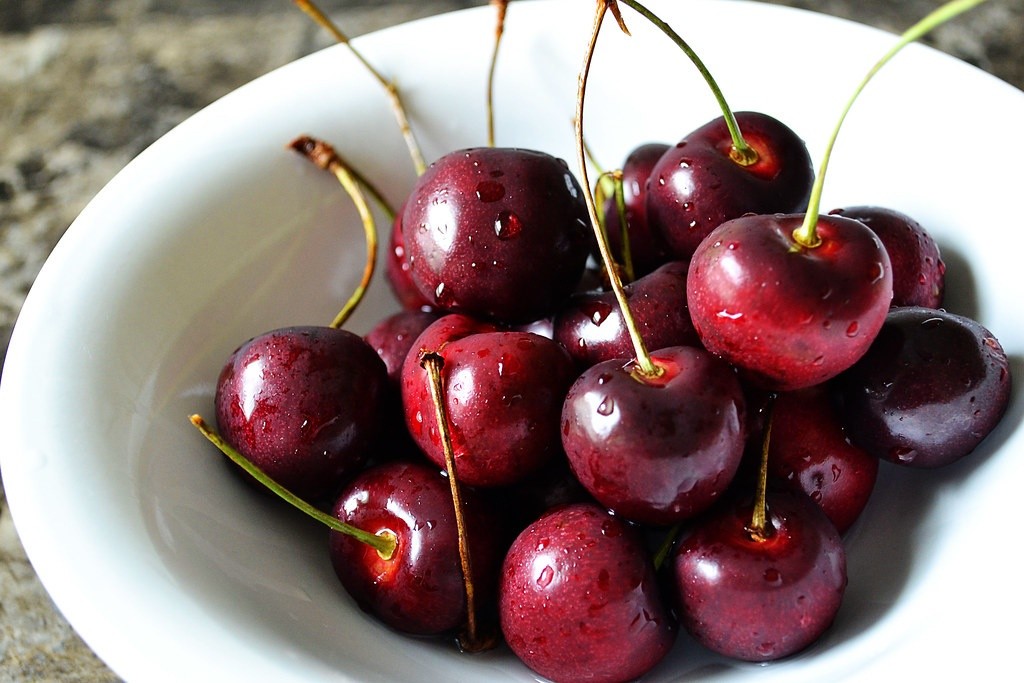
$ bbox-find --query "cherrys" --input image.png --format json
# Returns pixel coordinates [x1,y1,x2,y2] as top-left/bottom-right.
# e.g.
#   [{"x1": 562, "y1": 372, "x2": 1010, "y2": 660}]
[{"x1": 190, "y1": 0, "x2": 1008, "y2": 683}]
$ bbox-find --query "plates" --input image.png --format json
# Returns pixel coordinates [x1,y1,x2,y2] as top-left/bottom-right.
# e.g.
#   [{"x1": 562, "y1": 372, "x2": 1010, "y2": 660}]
[{"x1": 0, "y1": 0, "x2": 1024, "y2": 683}]
